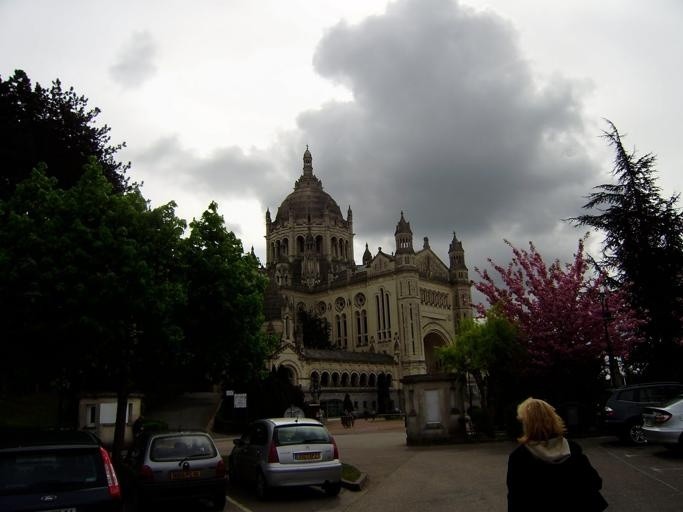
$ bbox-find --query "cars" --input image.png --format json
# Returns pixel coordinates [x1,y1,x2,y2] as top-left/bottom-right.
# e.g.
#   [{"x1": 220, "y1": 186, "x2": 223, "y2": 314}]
[
  {"x1": 138, "y1": 428, "x2": 226, "y2": 509},
  {"x1": 231, "y1": 417, "x2": 342, "y2": 495},
  {"x1": 642, "y1": 399, "x2": 683, "y2": 446}
]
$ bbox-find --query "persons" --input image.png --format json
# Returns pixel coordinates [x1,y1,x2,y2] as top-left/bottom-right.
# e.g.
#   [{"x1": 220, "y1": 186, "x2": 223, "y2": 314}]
[
  {"x1": 364, "y1": 409, "x2": 370, "y2": 420},
  {"x1": 507, "y1": 398, "x2": 608, "y2": 512},
  {"x1": 371, "y1": 409, "x2": 377, "y2": 420},
  {"x1": 132, "y1": 414, "x2": 145, "y2": 443},
  {"x1": 344, "y1": 407, "x2": 351, "y2": 416}
]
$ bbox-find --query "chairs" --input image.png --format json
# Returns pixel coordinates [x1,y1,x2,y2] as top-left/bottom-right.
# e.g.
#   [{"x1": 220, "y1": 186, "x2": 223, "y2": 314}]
[
  {"x1": 291, "y1": 429, "x2": 317, "y2": 442},
  {"x1": 156, "y1": 442, "x2": 203, "y2": 458}
]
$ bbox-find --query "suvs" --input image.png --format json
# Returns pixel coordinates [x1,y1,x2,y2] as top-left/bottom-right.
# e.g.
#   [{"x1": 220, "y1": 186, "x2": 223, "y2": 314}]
[
  {"x1": 1, "y1": 429, "x2": 120, "y2": 511},
  {"x1": 594, "y1": 384, "x2": 683, "y2": 446}
]
